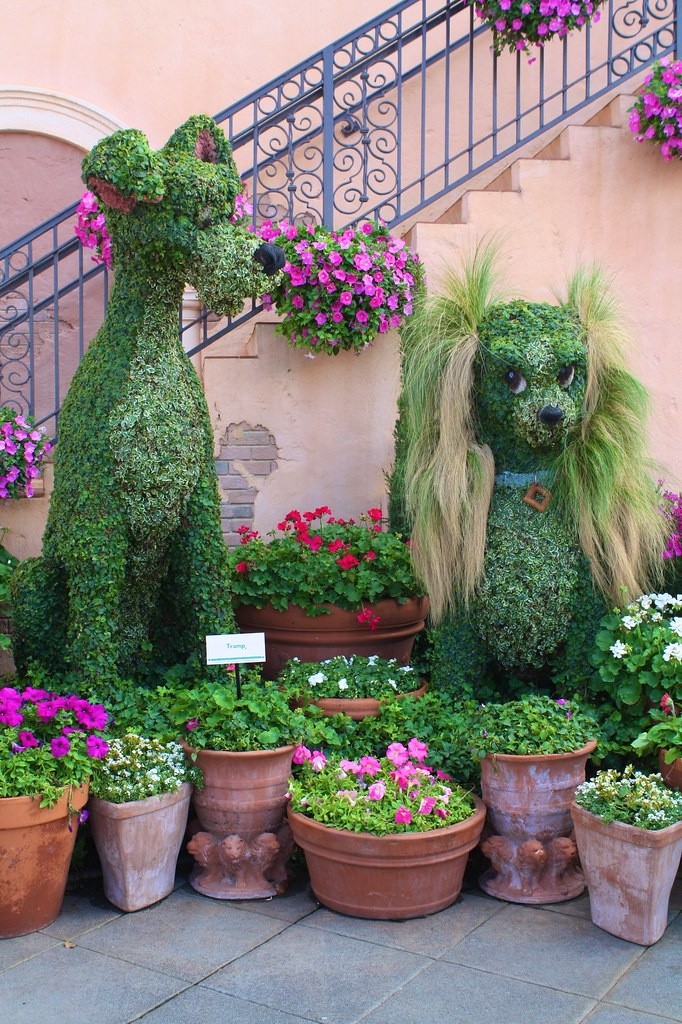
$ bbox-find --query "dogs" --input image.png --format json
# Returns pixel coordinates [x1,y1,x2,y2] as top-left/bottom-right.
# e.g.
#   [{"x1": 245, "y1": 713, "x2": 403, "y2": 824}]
[
  {"x1": 10, "y1": 112, "x2": 286, "y2": 693},
  {"x1": 396, "y1": 240, "x2": 671, "y2": 702}
]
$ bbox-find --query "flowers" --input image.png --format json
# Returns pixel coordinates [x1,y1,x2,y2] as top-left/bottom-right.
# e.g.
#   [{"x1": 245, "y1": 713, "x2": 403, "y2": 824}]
[{"x1": 0, "y1": 2, "x2": 682, "y2": 844}]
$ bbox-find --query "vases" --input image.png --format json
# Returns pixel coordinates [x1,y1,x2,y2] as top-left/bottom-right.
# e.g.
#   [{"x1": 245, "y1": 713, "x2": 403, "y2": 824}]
[
  {"x1": 95, "y1": 778, "x2": 198, "y2": 910},
  {"x1": 278, "y1": 675, "x2": 426, "y2": 723},
  {"x1": 485, "y1": 734, "x2": 597, "y2": 904},
  {"x1": 185, "y1": 737, "x2": 298, "y2": 900},
  {"x1": 0, "y1": 769, "x2": 91, "y2": 943},
  {"x1": 655, "y1": 738, "x2": 681, "y2": 785},
  {"x1": 289, "y1": 795, "x2": 487, "y2": 919},
  {"x1": 233, "y1": 588, "x2": 433, "y2": 678},
  {"x1": 573, "y1": 803, "x2": 681, "y2": 947}
]
